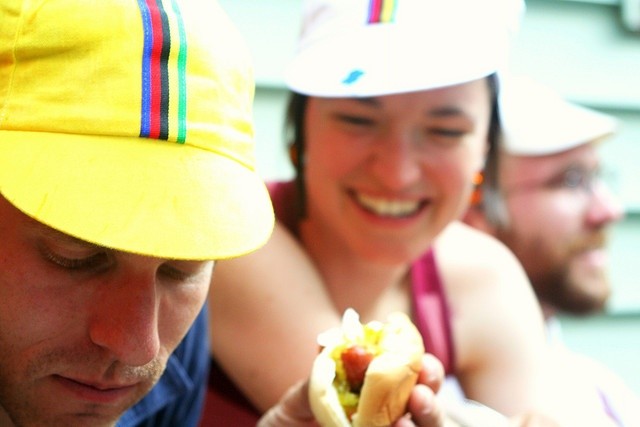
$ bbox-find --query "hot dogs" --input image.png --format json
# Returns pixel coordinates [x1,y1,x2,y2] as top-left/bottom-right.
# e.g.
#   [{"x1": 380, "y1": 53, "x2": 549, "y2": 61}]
[{"x1": 307, "y1": 306, "x2": 425, "y2": 426}]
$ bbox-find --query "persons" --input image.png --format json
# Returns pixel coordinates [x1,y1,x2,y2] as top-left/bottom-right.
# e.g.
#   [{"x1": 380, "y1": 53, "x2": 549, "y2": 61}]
[
  {"x1": 1, "y1": 0, "x2": 446, "y2": 425},
  {"x1": 197, "y1": 0, "x2": 552, "y2": 424},
  {"x1": 461, "y1": 82, "x2": 632, "y2": 424}
]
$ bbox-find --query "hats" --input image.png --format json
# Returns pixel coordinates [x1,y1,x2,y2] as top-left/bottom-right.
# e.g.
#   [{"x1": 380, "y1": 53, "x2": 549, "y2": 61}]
[
  {"x1": 286, "y1": 0, "x2": 526, "y2": 99},
  {"x1": 0, "y1": 2, "x2": 275, "y2": 261},
  {"x1": 495, "y1": 71, "x2": 617, "y2": 157}
]
